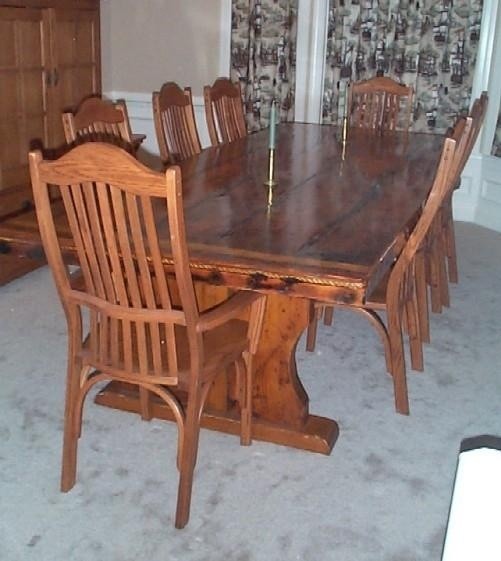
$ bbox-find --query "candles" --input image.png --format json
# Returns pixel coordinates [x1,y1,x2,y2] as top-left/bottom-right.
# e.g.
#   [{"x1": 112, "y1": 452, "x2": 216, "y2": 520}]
[{"x1": 267, "y1": 100, "x2": 277, "y2": 150}]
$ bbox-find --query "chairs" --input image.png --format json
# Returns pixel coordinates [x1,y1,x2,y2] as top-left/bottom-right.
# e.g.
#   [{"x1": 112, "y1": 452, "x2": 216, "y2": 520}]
[
  {"x1": 199, "y1": 78, "x2": 250, "y2": 148},
  {"x1": 150, "y1": 80, "x2": 204, "y2": 176},
  {"x1": 26, "y1": 138, "x2": 272, "y2": 531},
  {"x1": 60, "y1": 91, "x2": 141, "y2": 173},
  {"x1": 344, "y1": 77, "x2": 417, "y2": 135}
]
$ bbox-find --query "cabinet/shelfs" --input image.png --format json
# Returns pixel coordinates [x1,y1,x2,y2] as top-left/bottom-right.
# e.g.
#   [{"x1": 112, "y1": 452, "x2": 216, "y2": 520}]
[{"x1": 0, "y1": 0, "x2": 102, "y2": 288}]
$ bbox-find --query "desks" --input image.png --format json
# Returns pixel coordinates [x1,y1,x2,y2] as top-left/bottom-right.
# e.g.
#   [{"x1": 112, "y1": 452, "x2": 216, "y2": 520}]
[{"x1": 0, "y1": 114, "x2": 455, "y2": 455}]
